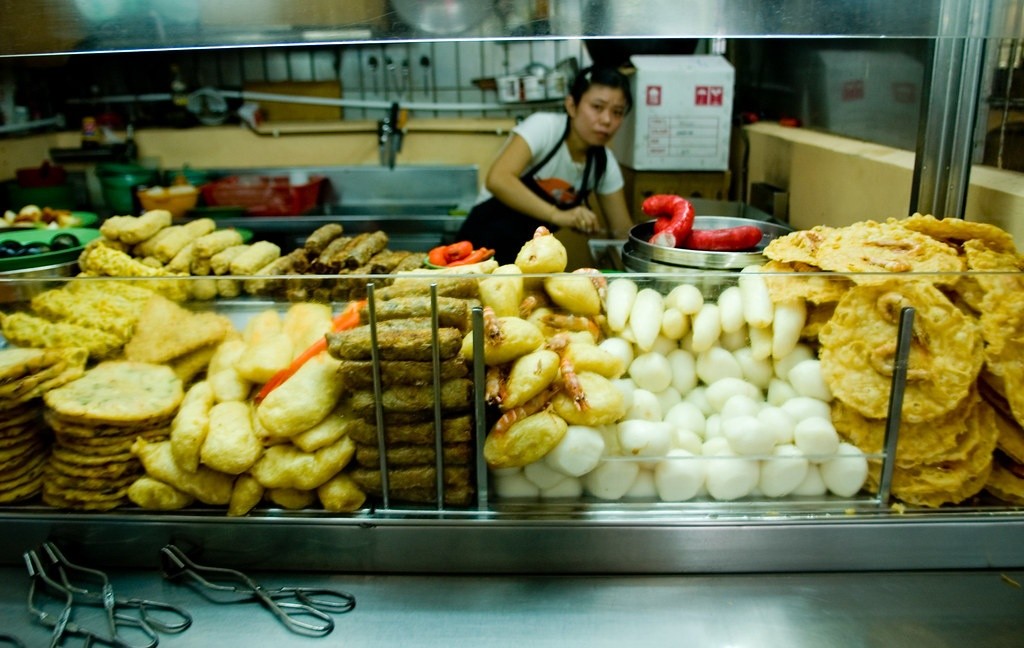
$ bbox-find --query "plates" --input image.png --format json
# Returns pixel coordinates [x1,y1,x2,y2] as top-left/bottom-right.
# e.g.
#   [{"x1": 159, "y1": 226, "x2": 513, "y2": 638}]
[{"x1": 188, "y1": 205, "x2": 247, "y2": 217}]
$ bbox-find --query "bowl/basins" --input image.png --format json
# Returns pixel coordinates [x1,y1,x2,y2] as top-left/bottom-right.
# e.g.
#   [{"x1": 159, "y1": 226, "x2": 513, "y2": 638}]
[
  {"x1": 0, "y1": 210, "x2": 100, "y2": 228},
  {"x1": 0, "y1": 259, "x2": 79, "y2": 304},
  {"x1": 136, "y1": 188, "x2": 203, "y2": 221},
  {"x1": 0, "y1": 228, "x2": 101, "y2": 271}
]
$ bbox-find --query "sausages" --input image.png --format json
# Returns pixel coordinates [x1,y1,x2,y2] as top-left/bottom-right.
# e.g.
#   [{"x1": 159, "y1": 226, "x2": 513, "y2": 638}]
[{"x1": 641, "y1": 194, "x2": 763, "y2": 250}]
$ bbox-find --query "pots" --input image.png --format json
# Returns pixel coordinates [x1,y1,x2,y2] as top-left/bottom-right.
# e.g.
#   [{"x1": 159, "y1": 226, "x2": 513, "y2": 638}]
[{"x1": 621, "y1": 216, "x2": 795, "y2": 306}]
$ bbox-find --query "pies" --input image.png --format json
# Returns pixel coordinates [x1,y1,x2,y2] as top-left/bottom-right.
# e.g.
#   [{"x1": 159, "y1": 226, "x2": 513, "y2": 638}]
[{"x1": 0, "y1": 345, "x2": 185, "y2": 508}]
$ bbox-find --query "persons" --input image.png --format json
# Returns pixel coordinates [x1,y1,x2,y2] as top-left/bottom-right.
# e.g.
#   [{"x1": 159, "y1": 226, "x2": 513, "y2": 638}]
[{"x1": 440, "y1": 61, "x2": 636, "y2": 267}]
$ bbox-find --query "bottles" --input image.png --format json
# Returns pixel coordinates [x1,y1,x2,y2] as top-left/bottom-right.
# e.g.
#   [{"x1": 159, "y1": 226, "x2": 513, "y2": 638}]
[{"x1": 81, "y1": 116, "x2": 102, "y2": 148}]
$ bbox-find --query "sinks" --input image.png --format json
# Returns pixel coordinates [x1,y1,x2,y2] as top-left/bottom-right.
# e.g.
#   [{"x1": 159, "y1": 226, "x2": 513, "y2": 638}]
[{"x1": 326, "y1": 165, "x2": 478, "y2": 235}]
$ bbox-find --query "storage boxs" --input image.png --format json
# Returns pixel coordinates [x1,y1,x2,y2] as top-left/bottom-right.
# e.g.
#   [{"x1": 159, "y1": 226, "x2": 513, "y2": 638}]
[
  {"x1": 607, "y1": 54, "x2": 736, "y2": 222},
  {"x1": 800, "y1": 53, "x2": 924, "y2": 154},
  {"x1": 199, "y1": 174, "x2": 325, "y2": 215}
]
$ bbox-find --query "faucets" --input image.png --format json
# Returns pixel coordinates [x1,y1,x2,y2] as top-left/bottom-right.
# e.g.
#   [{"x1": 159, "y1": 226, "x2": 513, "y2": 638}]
[{"x1": 376, "y1": 103, "x2": 404, "y2": 170}]
